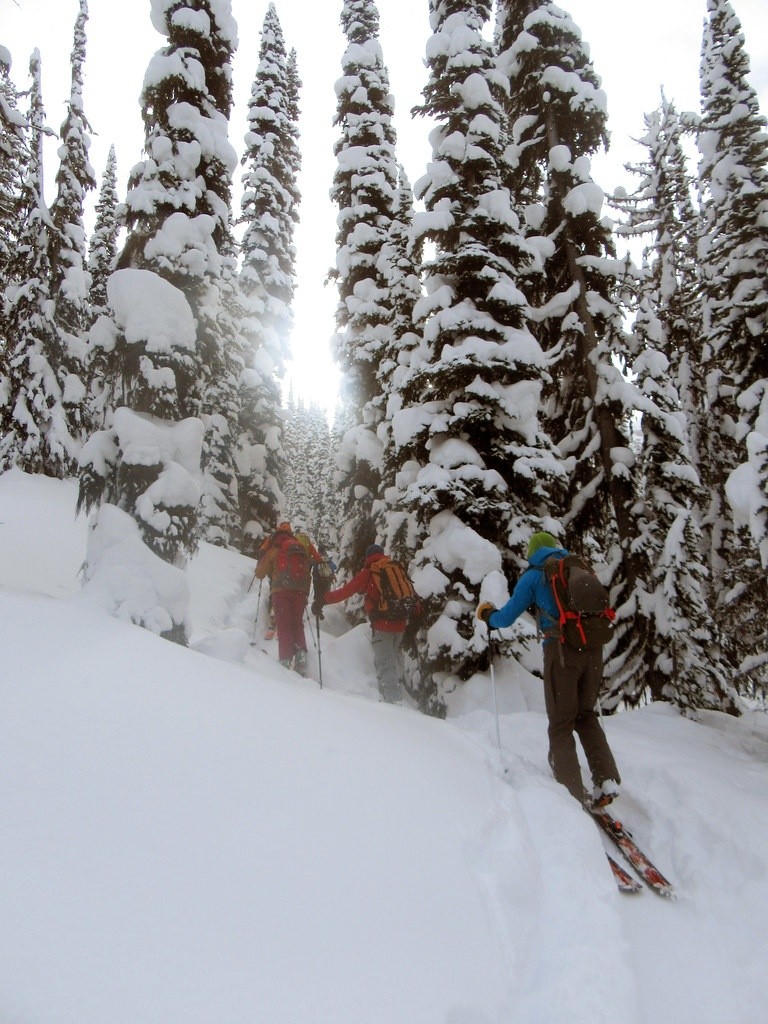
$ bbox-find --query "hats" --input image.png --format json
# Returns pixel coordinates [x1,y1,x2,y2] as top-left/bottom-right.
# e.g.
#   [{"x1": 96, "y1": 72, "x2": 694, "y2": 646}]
[
  {"x1": 527, "y1": 533, "x2": 555, "y2": 558},
  {"x1": 366, "y1": 544, "x2": 384, "y2": 559},
  {"x1": 277, "y1": 518, "x2": 291, "y2": 527}
]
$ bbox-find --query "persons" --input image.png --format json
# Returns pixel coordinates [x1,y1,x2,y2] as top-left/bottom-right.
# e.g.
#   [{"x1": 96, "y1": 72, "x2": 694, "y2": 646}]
[
  {"x1": 255, "y1": 521, "x2": 332, "y2": 675},
  {"x1": 477, "y1": 532, "x2": 621, "y2": 809},
  {"x1": 311, "y1": 545, "x2": 422, "y2": 705}
]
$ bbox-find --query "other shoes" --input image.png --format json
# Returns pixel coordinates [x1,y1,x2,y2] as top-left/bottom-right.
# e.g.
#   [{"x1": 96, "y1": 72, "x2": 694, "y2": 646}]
[
  {"x1": 264, "y1": 626, "x2": 276, "y2": 640},
  {"x1": 294, "y1": 649, "x2": 308, "y2": 674},
  {"x1": 280, "y1": 659, "x2": 292, "y2": 669},
  {"x1": 591, "y1": 777, "x2": 620, "y2": 809},
  {"x1": 553, "y1": 764, "x2": 584, "y2": 798},
  {"x1": 311, "y1": 596, "x2": 326, "y2": 615}
]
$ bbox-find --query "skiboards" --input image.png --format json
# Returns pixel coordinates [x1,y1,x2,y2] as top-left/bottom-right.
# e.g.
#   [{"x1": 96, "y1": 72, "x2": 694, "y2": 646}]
[{"x1": 576, "y1": 802, "x2": 680, "y2": 900}]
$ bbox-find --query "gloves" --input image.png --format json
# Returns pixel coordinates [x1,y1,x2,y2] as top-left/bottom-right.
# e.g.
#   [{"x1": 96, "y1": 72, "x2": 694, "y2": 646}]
[{"x1": 476, "y1": 600, "x2": 496, "y2": 620}]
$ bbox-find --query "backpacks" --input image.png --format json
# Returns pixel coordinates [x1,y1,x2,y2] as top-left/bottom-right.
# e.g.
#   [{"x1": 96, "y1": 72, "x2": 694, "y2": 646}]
[
  {"x1": 531, "y1": 555, "x2": 615, "y2": 652},
  {"x1": 279, "y1": 538, "x2": 308, "y2": 591},
  {"x1": 317, "y1": 553, "x2": 333, "y2": 580},
  {"x1": 371, "y1": 557, "x2": 417, "y2": 618}
]
